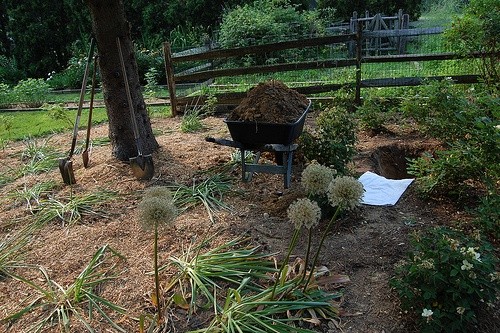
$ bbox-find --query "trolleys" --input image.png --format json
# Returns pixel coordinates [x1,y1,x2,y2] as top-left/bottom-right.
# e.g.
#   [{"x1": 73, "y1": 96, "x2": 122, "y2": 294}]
[{"x1": 206, "y1": 97, "x2": 311, "y2": 188}]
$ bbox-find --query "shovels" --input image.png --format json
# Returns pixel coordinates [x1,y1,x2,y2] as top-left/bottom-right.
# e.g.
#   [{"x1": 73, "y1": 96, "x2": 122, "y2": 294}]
[
  {"x1": 82, "y1": 55, "x2": 99, "y2": 169},
  {"x1": 57, "y1": 37, "x2": 96, "y2": 185}
]
